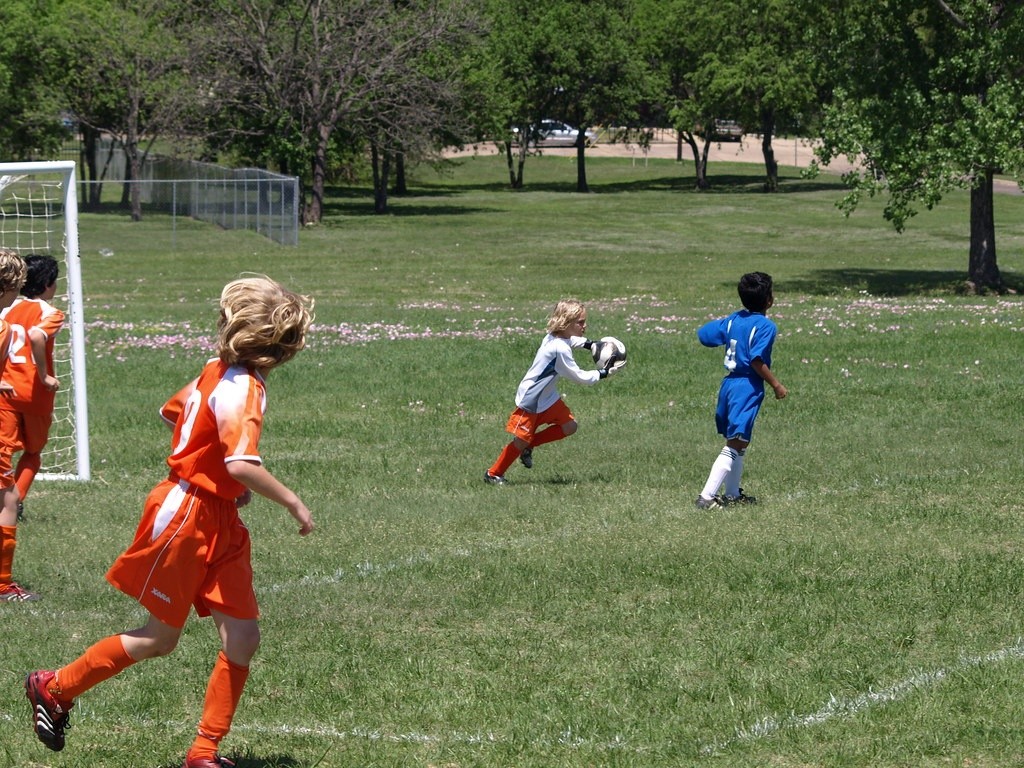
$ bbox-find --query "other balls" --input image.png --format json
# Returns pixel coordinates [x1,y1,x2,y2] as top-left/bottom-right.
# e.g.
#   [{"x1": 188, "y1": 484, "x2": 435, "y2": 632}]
[{"x1": 591, "y1": 336, "x2": 626, "y2": 371}]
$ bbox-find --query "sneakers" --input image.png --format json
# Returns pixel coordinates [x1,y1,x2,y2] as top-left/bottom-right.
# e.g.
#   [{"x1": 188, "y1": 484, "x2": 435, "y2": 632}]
[
  {"x1": 720, "y1": 488, "x2": 757, "y2": 505},
  {"x1": 514, "y1": 436, "x2": 534, "y2": 469},
  {"x1": 182, "y1": 750, "x2": 235, "y2": 768},
  {"x1": 695, "y1": 494, "x2": 724, "y2": 511},
  {"x1": 23, "y1": 668, "x2": 71, "y2": 753},
  {"x1": 484, "y1": 468, "x2": 510, "y2": 488},
  {"x1": 0, "y1": 583, "x2": 38, "y2": 603}
]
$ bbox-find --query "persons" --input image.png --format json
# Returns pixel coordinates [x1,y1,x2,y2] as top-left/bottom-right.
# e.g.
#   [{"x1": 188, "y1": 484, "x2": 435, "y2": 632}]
[
  {"x1": 25, "y1": 278, "x2": 315, "y2": 768},
  {"x1": 0, "y1": 250, "x2": 65, "y2": 603},
  {"x1": 697, "y1": 272, "x2": 788, "y2": 511},
  {"x1": 484, "y1": 299, "x2": 627, "y2": 486}
]
